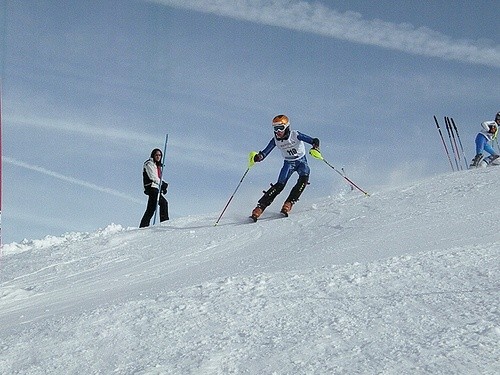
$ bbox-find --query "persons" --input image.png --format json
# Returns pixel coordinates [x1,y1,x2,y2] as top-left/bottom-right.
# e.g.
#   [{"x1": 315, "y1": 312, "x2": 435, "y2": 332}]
[
  {"x1": 248, "y1": 115, "x2": 320, "y2": 223},
  {"x1": 469, "y1": 112, "x2": 500, "y2": 168},
  {"x1": 138, "y1": 149, "x2": 169, "y2": 228}
]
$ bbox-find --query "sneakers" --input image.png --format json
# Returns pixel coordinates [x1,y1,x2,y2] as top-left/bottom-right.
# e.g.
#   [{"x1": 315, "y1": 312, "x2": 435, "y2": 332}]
[
  {"x1": 280, "y1": 201, "x2": 293, "y2": 217},
  {"x1": 249, "y1": 207, "x2": 263, "y2": 222}
]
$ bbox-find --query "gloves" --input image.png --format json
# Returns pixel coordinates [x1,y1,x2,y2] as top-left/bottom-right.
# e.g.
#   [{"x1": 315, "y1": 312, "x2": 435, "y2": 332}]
[
  {"x1": 254, "y1": 154, "x2": 264, "y2": 163},
  {"x1": 159, "y1": 181, "x2": 169, "y2": 194}
]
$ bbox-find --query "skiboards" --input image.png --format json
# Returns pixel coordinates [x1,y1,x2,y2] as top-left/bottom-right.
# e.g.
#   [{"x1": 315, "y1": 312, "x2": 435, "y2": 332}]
[
  {"x1": 251, "y1": 209, "x2": 289, "y2": 223},
  {"x1": 469, "y1": 162, "x2": 499, "y2": 168}
]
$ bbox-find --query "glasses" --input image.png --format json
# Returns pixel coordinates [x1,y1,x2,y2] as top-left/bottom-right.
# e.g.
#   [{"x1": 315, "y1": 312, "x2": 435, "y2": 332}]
[
  {"x1": 496, "y1": 115, "x2": 500, "y2": 120},
  {"x1": 273, "y1": 122, "x2": 290, "y2": 132}
]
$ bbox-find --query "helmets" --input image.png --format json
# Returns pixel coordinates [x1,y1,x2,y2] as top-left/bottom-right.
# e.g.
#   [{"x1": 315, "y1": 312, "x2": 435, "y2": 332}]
[{"x1": 271, "y1": 114, "x2": 289, "y2": 126}]
